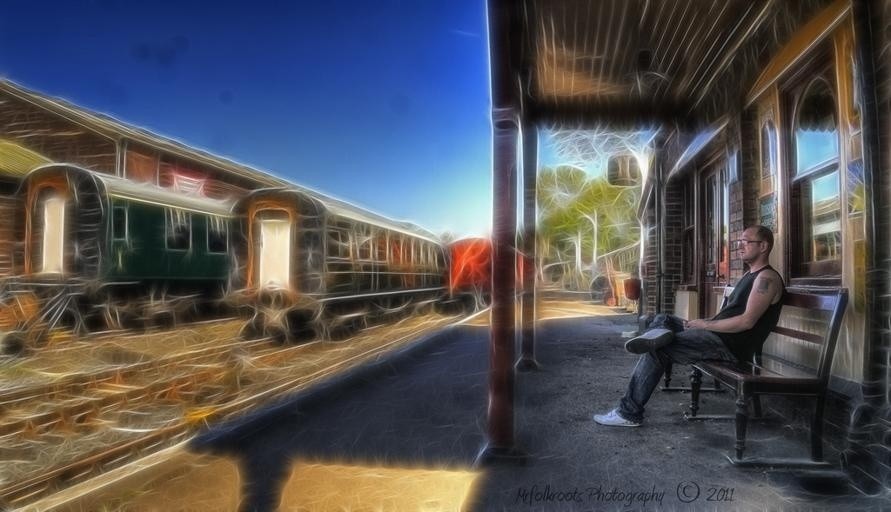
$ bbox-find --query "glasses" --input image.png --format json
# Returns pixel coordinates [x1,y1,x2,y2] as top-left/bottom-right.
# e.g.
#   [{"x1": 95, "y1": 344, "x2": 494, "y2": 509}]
[{"x1": 734, "y1": 238, "x2": 761, "y2": 249}]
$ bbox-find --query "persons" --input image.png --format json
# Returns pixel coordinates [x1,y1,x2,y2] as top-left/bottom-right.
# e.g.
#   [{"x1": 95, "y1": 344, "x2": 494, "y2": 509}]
[{"x1": 593, "y1": 225, "x2": 786, "y2": 427}]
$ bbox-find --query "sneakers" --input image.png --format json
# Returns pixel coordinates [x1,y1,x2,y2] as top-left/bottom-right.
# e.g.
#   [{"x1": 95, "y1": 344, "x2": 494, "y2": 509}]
[
  {"x1": 593, "y1": 408, "x2": 642, "y2": 428},
  {"x1": 624, "y1": 326, "x2": 674, "y2": 354}
]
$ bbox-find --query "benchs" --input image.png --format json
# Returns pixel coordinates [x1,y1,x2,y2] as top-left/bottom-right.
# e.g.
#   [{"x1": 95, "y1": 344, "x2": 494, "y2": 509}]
[{"x1": 655, "y1": 282, "x2": 856, "y2": 469}]
